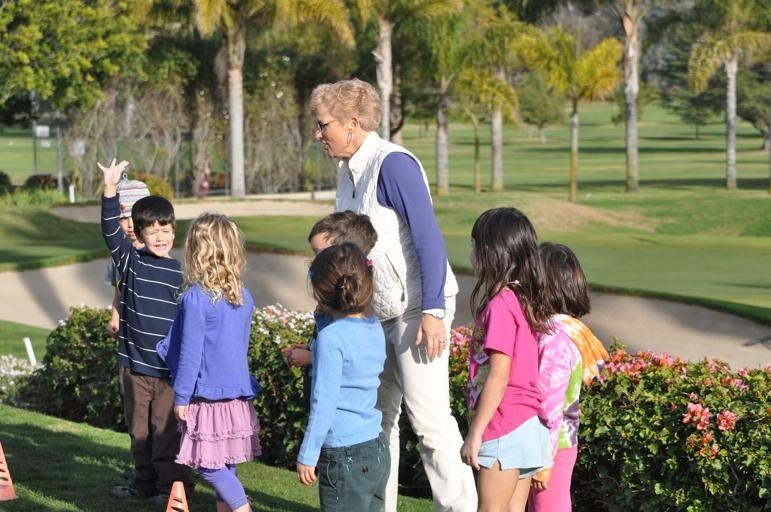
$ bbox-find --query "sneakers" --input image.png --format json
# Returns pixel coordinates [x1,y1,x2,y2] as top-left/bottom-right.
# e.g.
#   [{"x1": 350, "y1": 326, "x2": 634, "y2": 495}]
[{"x1": 111, "y1": 480, "x2": 192, "y2": 511}]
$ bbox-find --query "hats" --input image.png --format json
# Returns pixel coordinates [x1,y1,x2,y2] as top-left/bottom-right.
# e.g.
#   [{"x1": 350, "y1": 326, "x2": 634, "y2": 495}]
[{"x1": 115, "y1": 173, "x2": 150, "y2": 218}]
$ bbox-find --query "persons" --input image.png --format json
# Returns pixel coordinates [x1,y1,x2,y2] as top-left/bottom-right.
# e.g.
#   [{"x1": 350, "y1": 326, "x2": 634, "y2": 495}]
[
  {"x1": 97, "y1": 158, "x2": 196, "y2": 506},
  {"x1": 460, "y1": 208, "x2": 554, "y2": 511},
  {"x1": 309, "y1": 78, "x2": 478, "y2": 512},
  {"x1": 525, "y1": 241, "x2": 610, "y2": 512},
  {"x1": 103, "y1": 173, "x2": 151, "y2": 495},
  {"x1": 156, "y1": 213, "x2": 262, "y2": 510},
  {"x1": 281, "y1": 210, "x2": 391, "y2": 512}
]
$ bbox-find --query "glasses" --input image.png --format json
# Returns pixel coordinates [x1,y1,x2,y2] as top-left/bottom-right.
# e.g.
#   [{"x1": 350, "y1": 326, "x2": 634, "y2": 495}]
[{"x1": 317, "y1": 119, "x2": 339, "y2": 128}]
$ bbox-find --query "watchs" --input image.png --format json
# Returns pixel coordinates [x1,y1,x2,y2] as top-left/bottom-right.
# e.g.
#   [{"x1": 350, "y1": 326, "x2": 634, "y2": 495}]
[{"x1": 422, "y1": 309, "x2": 446, "y2": 319}]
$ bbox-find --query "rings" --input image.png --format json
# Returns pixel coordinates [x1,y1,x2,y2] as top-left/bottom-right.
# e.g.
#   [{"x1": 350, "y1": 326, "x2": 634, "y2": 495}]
[{"x1": 440, "y1": 341, "x2": 445, "y2": 343}]
